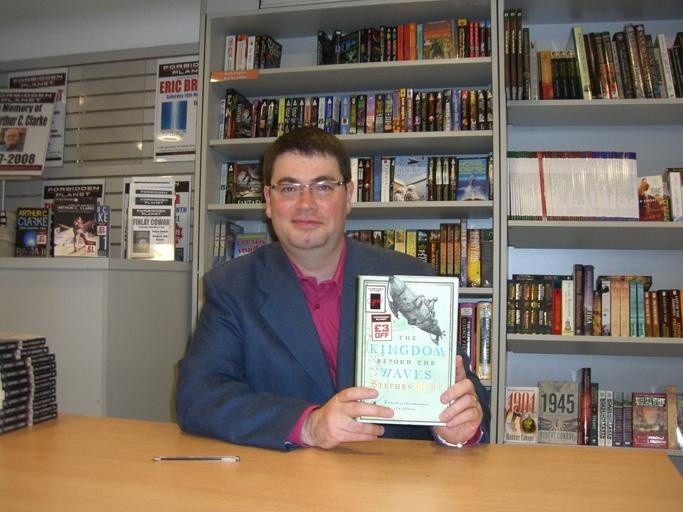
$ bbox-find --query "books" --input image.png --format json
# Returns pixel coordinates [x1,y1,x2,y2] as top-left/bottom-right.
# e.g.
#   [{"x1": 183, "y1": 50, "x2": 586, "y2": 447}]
[
  {"x1": 54, "y1": 202, "x2": 110, "y2": 260},
  {"x1": 213, "y1": 218, "x2": 268, "y2": 266},
  {"x1": 460, "y1": 302, "x2": 476, "y2": 379},
  {"x1": 507, "y1": 263, "x2": 683, "y2": 338},
  {"x1": 477, "y1": 303, "x2": 492, "y2": 380},
  {"x1": 220, "y1": 156, "x2": 495, "y2": 205},
  {"x1": 505, "y1": 386, "x2": 538, "y2": 443},
  {"x1": 631, "y1": 393, "x2": 668, "y2": 449},
  {"x1": 354, "y1": 275, "x2": 458, "y2": 427},
  {"x1": 127, "y1": 176, "x2": 176, "y2": 263},
  {"x1": 666, "y1": 385, "x2": 683, "y2": 449},
  {"x1": 504, "y1": 8, "x2": 683, "y2": 100},
  {"x1": 576, "y1": 368, "x2": 632, "y2": 448},
  {"x1": 508, "y1": 152, "x2": 682, "y2": 221},
  {"x1": 346, "y1": 218, "x2": 494, "y2": 290},
  {"x1": 217, "y1": 88, "x2": 494, "y2": 140},
  {"x1": 538, "y1": 381, "x2": 579, "y2": 444},
  {"x1": 1, "y1": 333, "x2": 57, "y2": 434},
  {"x1": 0, "y1": 89, "x2": 58, "y2": 177},
  {"x1": 223, "y1": 18, "x2": 492, "y2": 71},
  {"x1": 14, "y1": 207, "x2": 51, "y2": 258}
]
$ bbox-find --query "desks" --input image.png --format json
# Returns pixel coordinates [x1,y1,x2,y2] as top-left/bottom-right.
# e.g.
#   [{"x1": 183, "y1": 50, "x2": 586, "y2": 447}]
[{"x1": 0, "y1": 411, "x2": 683, "y2": 512}]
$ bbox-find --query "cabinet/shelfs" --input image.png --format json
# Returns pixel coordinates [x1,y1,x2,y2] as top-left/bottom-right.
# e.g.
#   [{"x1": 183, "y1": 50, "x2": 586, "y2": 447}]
[
  {"x1": 498, "y1": 0, "x2": 683, "y2": 470},
  {"x1": 106, "y1": 259, "x2": 193, "y2": 422},
  {"x1": 198, "y1": 0, "x2": 497, "y2": 442},
  {"x1": 0, "y1": 258, "x2": 108, "y2": 416}
]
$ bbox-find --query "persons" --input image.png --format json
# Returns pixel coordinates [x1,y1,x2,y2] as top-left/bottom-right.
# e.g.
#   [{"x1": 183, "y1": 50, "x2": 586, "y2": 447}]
[{"x1": 173, "y1": 127, "x2": 490, "y2": 452}]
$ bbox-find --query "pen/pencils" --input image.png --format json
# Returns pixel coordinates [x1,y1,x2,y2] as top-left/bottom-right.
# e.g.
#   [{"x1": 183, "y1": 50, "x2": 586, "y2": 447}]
[{"x1": 152, "y1": 455, "x2": 240, "y2": 463}]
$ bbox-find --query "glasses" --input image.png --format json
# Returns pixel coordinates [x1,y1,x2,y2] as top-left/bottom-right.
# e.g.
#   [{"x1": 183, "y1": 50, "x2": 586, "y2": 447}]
[{"x1": 267, "y1": 181, "x2": 345, "y2": 197}]
